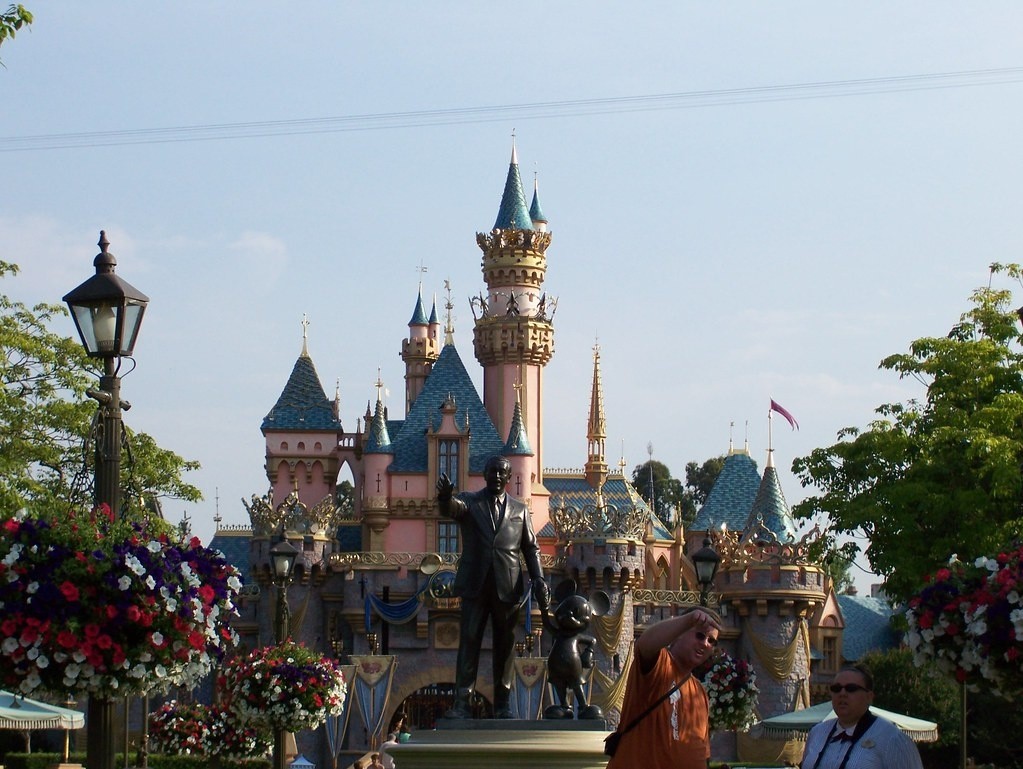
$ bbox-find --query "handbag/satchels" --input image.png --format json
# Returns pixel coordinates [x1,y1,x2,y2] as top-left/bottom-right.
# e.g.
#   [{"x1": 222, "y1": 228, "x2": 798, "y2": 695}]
[{"x1": 603, "y1": 731, "x2": 621, "y2": 758}]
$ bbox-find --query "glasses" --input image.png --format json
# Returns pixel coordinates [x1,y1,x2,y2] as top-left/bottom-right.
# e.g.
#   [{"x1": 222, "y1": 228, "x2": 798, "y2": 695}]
[
  {"x1": 830, "y1": 683, "x2": 869, "y2": 693},
  {"x1": 695, "y1": 632, "x2": 719, "y2": 647}
]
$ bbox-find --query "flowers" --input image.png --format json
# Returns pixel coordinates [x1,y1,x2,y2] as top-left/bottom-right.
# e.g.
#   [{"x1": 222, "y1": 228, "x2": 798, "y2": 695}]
[
  {"x1": 147, "y1": 701, "x2": 277, "y2": 764},
  {"x1": 0, "y1": 493, "x2": 244, "y2": 700},
  {"x1": 903, "y1": 549, "x2": 1023, "y2": 703},
  {"x1": 214, "y1": 636, "x2": 347, "y2": 733},
  {"x1": 691, "y1": 646, "x2": 761, "y2": 734}
]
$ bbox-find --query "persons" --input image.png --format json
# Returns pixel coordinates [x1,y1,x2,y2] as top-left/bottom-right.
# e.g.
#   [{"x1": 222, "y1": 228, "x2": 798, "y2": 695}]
[
  {"x1": 353, "y1": 760, "x2": 367, "y2": 769},
  {"x1": 366, "y1": 754, "x2": 385, "y2": 769},
  {"x1": 378, "y1": 733, "x2": 398, "y2": 769},
  {"x1": 801, "y1": 666, "x2": 923, "y2": 769},
  {"x1": 395, "y1": 724, "x2": 411, "y2": 744},
  {"x1": 438, "y1": 453, "x2": 551, "y2": 717},
  {"x1": 603, "y1": 605, "x2": 722, "y2": 769}
]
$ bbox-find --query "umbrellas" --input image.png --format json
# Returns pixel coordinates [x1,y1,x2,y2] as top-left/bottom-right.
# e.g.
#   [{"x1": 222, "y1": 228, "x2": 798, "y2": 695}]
[{"x1": 747, "y1": 700, "x2": 939, "y2": 743}]
